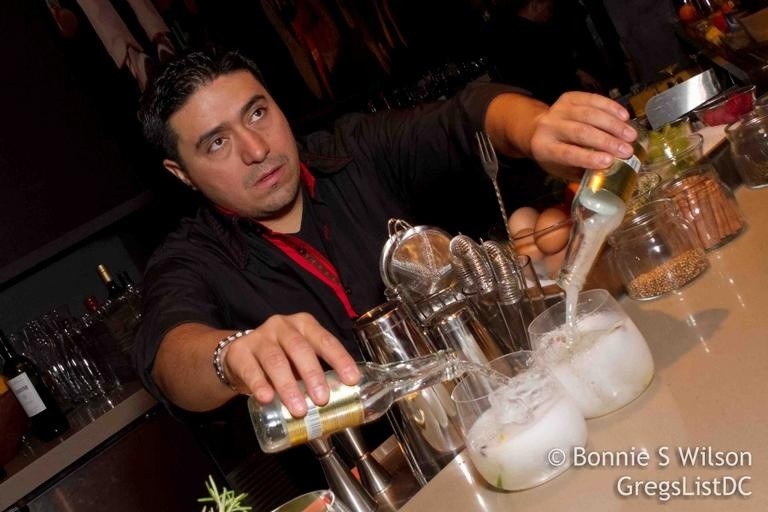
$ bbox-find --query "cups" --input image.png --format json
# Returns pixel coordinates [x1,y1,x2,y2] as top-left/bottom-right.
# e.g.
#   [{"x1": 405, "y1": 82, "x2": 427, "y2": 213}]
[
  {"x1": 353, "y1": 215, "x2": 582, "y2": 487},
  {"x1": 448, "y1": 286, "x2": 660, "y2": 493}
]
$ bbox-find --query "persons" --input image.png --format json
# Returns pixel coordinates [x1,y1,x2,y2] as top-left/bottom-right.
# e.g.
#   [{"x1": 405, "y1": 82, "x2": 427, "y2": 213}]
[
  {"x1": 492, "y1": 1, "x2": 605, "y2": 98},
  {"x1": 120, "y1": 38, "x2": 635, "y2": 492}
]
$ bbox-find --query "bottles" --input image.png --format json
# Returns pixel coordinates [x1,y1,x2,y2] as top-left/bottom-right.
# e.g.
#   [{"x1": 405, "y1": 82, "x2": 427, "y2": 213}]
[
  {"x1": 553, "y1": 118, "x2": 650, "y2": 291},
  {"x1": 609, "y1": 88, "x2": 768, "y2": 303},
  {"x1": 0, "y1": 263, "x2": 163, "y2": 463},
  {"x1": 247, "y1": 346, "x2": 462, "y2": 454}
]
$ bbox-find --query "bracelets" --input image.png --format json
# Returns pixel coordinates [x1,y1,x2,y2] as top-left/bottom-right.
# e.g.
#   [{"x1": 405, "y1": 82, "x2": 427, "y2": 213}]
[{"x1": 208, "y1": 323, "x2": 254, "y2": 396}]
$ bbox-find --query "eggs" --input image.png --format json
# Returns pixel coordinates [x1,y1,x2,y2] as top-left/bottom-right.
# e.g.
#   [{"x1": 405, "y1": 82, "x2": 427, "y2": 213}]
[{"x1": 505, "y1": 206, "x2": 572, "y2": 297}]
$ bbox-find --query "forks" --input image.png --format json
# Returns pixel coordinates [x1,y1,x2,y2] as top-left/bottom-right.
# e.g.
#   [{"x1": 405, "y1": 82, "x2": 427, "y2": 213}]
[{"x1": 473, "y1": 125, "x2": 527, "y2": 276}]
